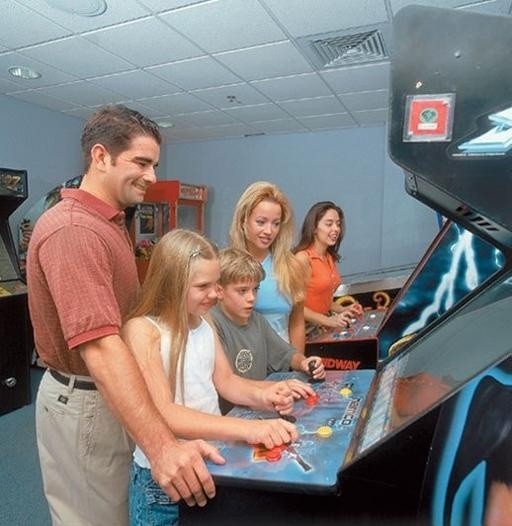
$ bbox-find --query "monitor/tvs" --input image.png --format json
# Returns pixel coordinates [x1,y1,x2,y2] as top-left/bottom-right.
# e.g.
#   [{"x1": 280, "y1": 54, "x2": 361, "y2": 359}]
[
  {"x1": 0, "y1": 229, "x2": 16, "y2": 275},
  {"x1": 389, "y1": 266, "x2": 512, "y2": 435}
]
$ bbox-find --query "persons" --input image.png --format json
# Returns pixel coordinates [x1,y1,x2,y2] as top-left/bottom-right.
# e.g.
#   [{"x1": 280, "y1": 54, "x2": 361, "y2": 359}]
[
  {"x1": 229, "y1": 182, "x2": 306, "y2": 357},
  {"x1": 26, "y1": 103, "x2": 225, "y2": 526},
  {"x1": 290, "y1": 201, "x2": 354, "y2": 328},
  {"x1": 214, "y1": 248, "x2": 327, "y2": 400},
  {"x1": 122, "y1": 229, "x2": 298, "y2": 526}
]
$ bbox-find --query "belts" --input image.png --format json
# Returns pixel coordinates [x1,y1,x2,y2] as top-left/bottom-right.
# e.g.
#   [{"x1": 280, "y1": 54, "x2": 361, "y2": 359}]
[{"x1": 49, "y1": 368, "x2": 98, "y2": 390}]
